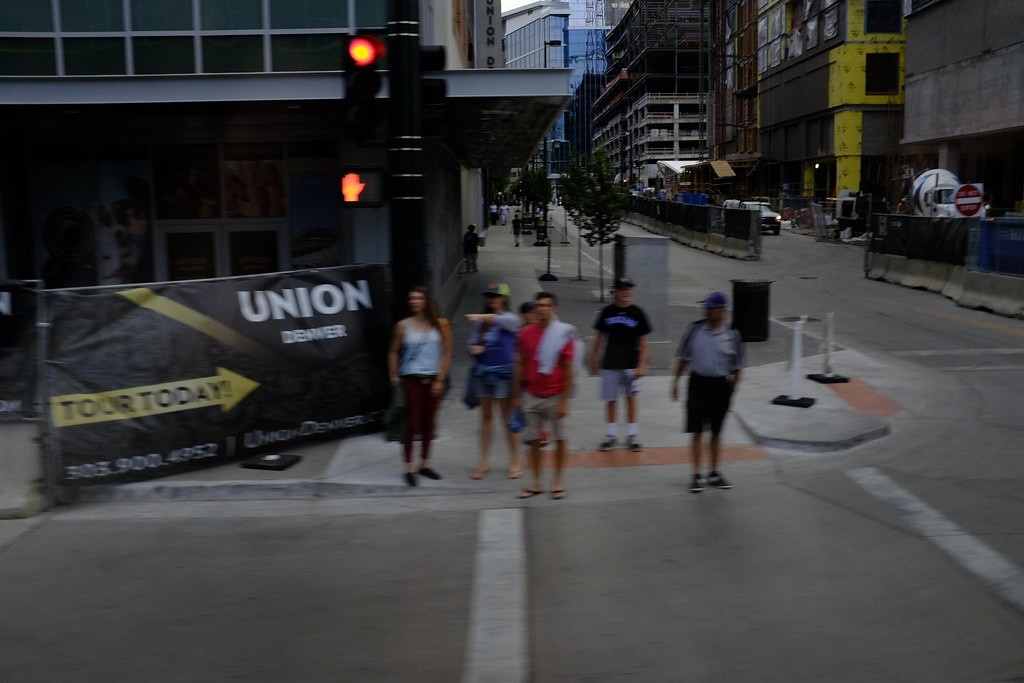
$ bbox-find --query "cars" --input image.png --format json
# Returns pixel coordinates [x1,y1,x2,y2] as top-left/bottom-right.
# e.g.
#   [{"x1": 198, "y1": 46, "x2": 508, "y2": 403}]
[{"x1": 739, "y1": 202, "x2": 782, "y2": 235}]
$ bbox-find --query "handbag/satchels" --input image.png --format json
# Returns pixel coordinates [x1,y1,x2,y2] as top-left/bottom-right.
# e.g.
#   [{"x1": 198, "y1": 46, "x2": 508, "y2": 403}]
[
  {"x1": 464, "y1": 367, "x2": 480, "y2": 408},
  {"x1": 507, "y1": 404, "x2": 526, "y2": 433},
  {"x1": 392, "y1": 380, "x2": 406, "y2": 414}
]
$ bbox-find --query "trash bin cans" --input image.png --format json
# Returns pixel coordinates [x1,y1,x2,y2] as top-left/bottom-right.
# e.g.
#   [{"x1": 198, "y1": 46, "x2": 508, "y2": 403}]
[{"x1": 729, "y1": 279, "x2": 774, "y2": 342}]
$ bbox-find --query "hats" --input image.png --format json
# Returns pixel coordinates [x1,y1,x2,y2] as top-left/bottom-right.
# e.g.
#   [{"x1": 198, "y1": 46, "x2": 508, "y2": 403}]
[
  {"x1": 481, "y1": 283, "x2": 510, "y2": 297},
  {"x1": 614, "y1": 277, "x2": 636, "y2": 288},
  {"x1": 696, "y1": 292, "x2": 727, "y2": 307},
  {"x1": 521, "y1": 301, "x2": 536, "y2": 313}
]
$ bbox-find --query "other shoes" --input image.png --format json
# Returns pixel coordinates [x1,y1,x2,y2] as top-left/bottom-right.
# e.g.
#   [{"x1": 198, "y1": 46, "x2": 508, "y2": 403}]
[
  {"x1": 420, "y1": 468, "x2": 440, "y2": 480},
  {"x1": 541, "y1": 427, "x2": 549, "y2": 445},
  {"x1": 406, "y1": 472, "x2": 416, "y2": 486}
]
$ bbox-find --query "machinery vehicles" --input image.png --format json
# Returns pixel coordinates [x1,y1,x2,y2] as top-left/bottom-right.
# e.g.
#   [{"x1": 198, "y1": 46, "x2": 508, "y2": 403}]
[{"x1": 904, "y1": 168, "x2": 963, "y2": 218}]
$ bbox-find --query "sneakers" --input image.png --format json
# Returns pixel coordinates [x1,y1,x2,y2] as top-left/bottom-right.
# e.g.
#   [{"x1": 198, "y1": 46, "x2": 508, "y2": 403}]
[
  {"x1": 601, "y1": 434, "x2": 616, "y2": 450},
  {"x1": 708, "y1": 471, "x2": 733, "y2": 488},
  {"x1": 627, "y1": 433, "x2": 640, "y2": 451},
  {"x1": 690, "y1": 474, "x2": 704, "y2": 491}
]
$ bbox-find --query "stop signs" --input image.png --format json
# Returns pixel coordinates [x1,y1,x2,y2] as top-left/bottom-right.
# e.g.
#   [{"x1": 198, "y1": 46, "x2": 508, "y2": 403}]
[{"x1": 952, "y1": 183, "x2": 985, "y2": 217}]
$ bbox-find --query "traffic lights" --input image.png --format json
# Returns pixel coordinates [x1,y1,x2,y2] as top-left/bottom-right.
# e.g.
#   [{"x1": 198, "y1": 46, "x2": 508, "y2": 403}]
[
  {"x1": 418, "y1": 45, "x2": 449, "y2": 140},
  {"x1": 337, "y1": 161, "x2": 383, "y2": 208},
  {"x1": 338, "y1": 35, "x2": 384, "y2": 141}
]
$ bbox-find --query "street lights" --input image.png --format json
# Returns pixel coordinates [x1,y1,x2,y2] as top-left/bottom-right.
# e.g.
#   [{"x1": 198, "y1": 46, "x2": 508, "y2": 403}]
[{"x1": 542, "y1": 39, "x2": 563, "y2": 244}]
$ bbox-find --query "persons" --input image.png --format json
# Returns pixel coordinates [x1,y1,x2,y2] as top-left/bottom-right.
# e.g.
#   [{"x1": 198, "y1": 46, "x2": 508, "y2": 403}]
[
  {"x1": 510, "y1": 214, "x2": 523, "y2": 247},
  {"x1": 462, "y1": 281, "x2": 575, "y2": 501},
  {"x1": 387, "y1": 286, "x2": 454, "y2": 487},
  {"x1": 488, "y1": 201, "x2": 510, "y2": 226},
  {"x1": 586, "y1": 277, "x2": 653, "y2": 453},
  {"x1": 670, "y1": 291, "x2": 748, "y2": 493},
  {"x1": 462, "y1": 224, "x2": 485, "y2": 272}
]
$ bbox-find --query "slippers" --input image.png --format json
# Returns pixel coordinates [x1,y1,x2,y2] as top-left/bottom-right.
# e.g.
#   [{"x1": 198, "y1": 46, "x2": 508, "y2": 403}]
[
  {"x1": 472, "y1": 469, "x2": 489, "y2": 480},
  {"x1": 508, "y1": 465, "x2": 522, "y2": 478},
  {"x1": 550, "y1": 490, "x2": 563, "y2": 499},
  {"x1": 518, "y1": 489, "x2": 543, "y2": 498}
]
